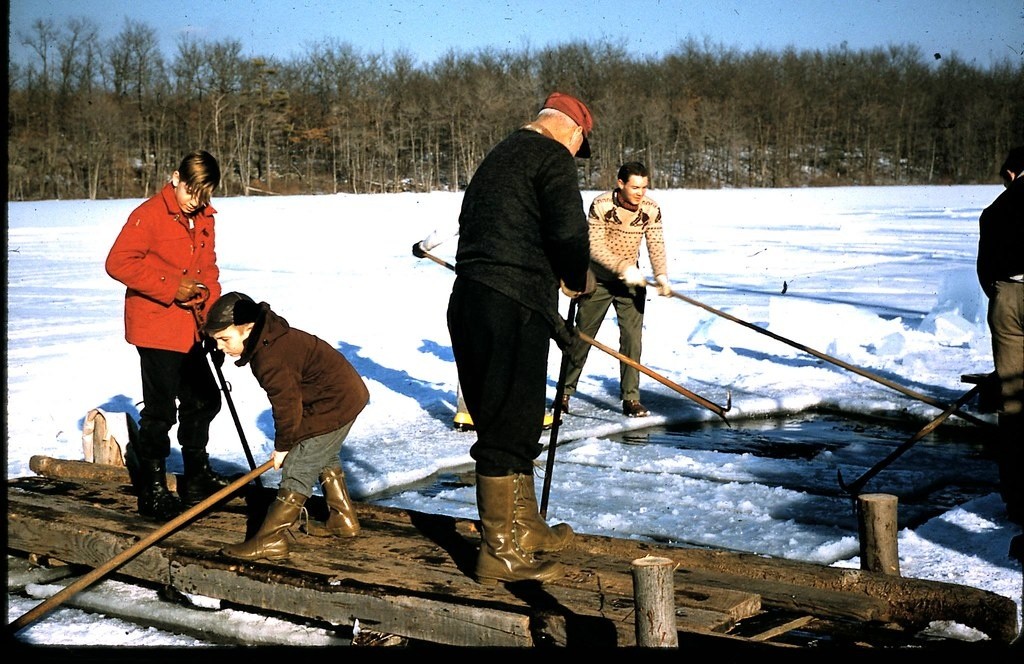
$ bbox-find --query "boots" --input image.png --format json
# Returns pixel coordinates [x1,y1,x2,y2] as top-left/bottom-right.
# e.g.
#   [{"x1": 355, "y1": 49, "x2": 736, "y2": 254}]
[
  {"x1": 181, "y1": 448, "x2": 227, "y2": 501},
  {"x1": 302, "y1": 466, "x2": 360, "y2": 537},
  {"x1": 138, "y1": 458, "x2": 181, "y2": 520},
  {"x1": 220, "y1": 490, "x2": 307, "y2": 561},
  {"x1": 474, "y1": 473, "x2": 564, "y2": 585},
  {"x1": 514, "y1": 475, "x2": 574, "y2": 553}
]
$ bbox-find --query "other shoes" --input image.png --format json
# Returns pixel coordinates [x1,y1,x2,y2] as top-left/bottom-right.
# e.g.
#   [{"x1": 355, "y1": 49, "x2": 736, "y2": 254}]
[
  {"x1": 453, "y1": 414, "x2": 476, "y2": 433},
  {"x1": 622, "y1": 400, "x2": 650, "y2": 417},
  {"x1": 550, "y1": 394, "x2": 568, "y2": 414},
  {"x1": 1010, "y1": 534, "x2": 1024, "y2": 565},
  {"x1": 542, "y1": 414, "x2": 562, "y2": 429}
]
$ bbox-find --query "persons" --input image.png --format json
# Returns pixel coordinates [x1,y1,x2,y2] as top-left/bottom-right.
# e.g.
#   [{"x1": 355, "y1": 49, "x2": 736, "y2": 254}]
[
  {"x1": 444, "y1": 89, "x2": 595, "y2": 585},
  {"x1": 104, "y1": 149, "x2": 246, "y2": 517},
  {"x1": 975, "y1": 143, "x2": 1024, "y2": 525},
  {"x1": 551, "y1": 161, "x2": 675, "y2": 418},
  {"x1": 204, "y1": 292, "x2": 371, "y2": 561}
]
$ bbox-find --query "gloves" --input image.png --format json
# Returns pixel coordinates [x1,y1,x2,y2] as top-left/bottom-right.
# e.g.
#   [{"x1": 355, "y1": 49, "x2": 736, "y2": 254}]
[
  {"x1": 623, "y1": 266, "x2": 646, "y2": 288},
  {"x1": 656, "y1": 274, "x2": 671, "y2": 298}
]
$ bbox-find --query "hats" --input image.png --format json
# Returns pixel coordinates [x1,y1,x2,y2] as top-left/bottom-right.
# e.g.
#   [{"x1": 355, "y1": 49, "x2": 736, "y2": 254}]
[{"x1": 539, "y1": 92, "x2": 593, "y2": 158}]
